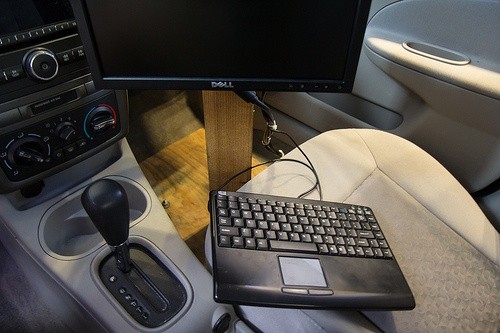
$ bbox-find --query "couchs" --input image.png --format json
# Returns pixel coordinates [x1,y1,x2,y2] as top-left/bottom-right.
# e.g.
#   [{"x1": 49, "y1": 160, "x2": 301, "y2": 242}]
[{"x1": 205, "y1": 128, "x2": 500, "y2": 333}]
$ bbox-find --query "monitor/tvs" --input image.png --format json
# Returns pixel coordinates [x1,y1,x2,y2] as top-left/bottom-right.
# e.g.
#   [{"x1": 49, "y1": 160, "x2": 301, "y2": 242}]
[{"x1": 70, "y1": 0, "x2": 372, "y2": 93}]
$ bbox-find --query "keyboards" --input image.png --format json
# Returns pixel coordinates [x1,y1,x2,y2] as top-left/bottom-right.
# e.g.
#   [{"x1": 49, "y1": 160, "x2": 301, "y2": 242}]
[{"x1": 210, "y1": 190, "x2": 417, "y2": 312}]
